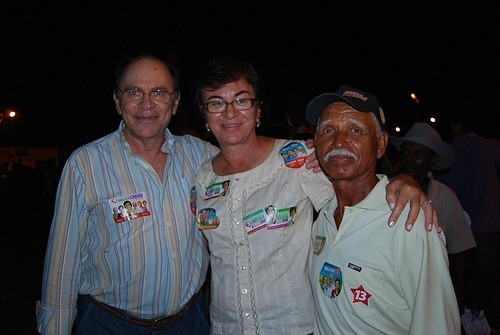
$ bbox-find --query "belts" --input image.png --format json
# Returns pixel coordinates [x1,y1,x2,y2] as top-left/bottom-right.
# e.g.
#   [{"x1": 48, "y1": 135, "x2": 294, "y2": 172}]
[{"x1": 91, "y1": 290, "x2": 200, "y2": 333}]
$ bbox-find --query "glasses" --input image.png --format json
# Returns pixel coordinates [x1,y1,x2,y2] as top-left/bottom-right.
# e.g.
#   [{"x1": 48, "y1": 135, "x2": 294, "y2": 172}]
[
  {"x1": 200, "y1": 97, "x2": 260, "y2": 114},
  {"x1": 116, "y1": 85, "x2": 174, "y2": 104}
]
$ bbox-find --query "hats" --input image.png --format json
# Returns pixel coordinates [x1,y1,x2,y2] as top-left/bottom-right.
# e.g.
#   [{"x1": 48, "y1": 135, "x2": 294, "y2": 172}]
[
  {"x1": 389, "y1": 123, "x2": 454, "y2": 169},
  {"x1": 305, "y1": 87, "x2": 385, "y2": 132}
]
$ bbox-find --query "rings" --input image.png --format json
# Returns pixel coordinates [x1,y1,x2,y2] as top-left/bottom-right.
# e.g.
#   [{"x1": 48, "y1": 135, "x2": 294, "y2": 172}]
[{"x1": 422, "y1": 199, "x2": 432, "y2": 208}]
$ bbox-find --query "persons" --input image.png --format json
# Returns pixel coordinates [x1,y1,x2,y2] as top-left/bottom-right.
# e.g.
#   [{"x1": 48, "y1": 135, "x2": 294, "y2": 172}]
[
  {"x1": 220, "y1": 181, "x2": 229, "y2": 196},
  {"x1": 375, "y1": 105, "x2": 500, "y2": 335},
  {"x1": 194, "y1": 59, "x2": 440, "y2": 335},
  {"x1": 0, "y1": 158, "x2": 64, "y2": 191},
  {"x1": 264, "y1": 205, "x2": 276, "y2": 223},
  {"x1": 245, "y1": 220, "x2": 255, "y2": 230},
  {"x1": 304, "y1": 84, "x2": 461, "y2": 335},
  {"x1": 288, "y1": 206, "x2": 296, "y2": 224},
  {"x1": 321, "y1": 273, "x2": 340, "y2": 298},
  {"x1": 281, "y1": 146, "x2": 306, "y2": 163},
  {"x1": 35, "y1": 52, "x2": 323, "y2": 335},
  {"x1": 112, "y1": 201, "x2": 148, "y2": 219}
]
